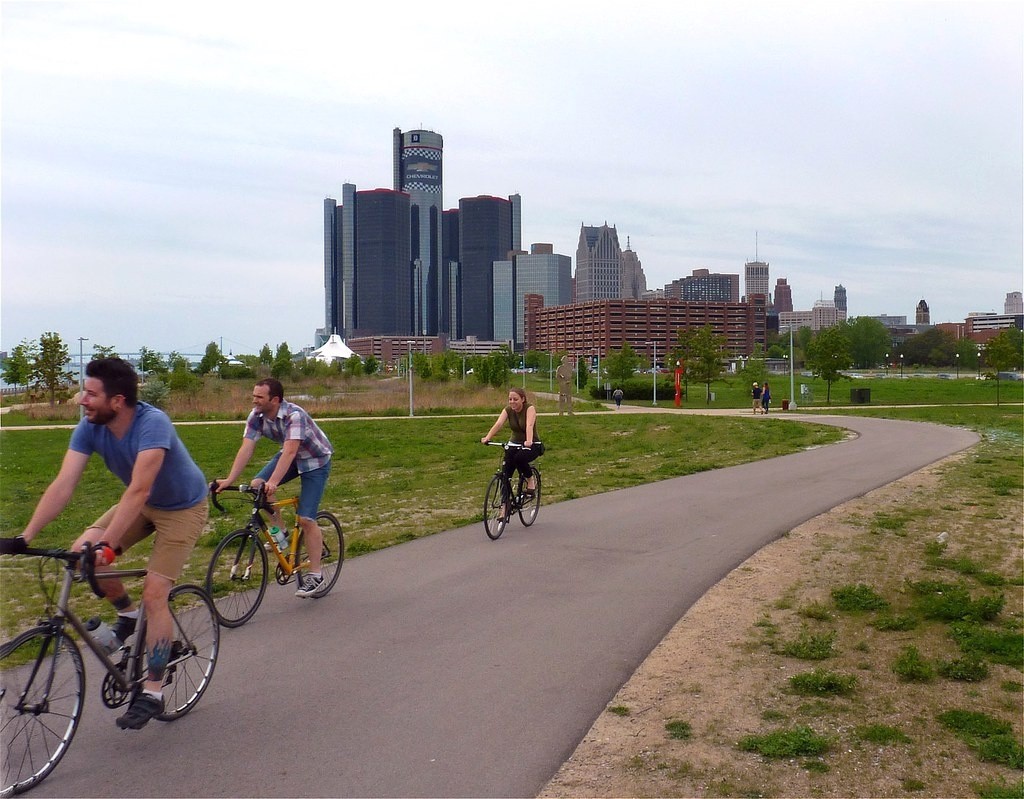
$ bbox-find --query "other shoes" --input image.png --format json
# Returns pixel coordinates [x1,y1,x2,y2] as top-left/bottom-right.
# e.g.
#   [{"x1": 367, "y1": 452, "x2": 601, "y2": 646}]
[
  {"x1": 525, "y1": 488, "x2": 536, "y2": 495},
  {"x1": 497, "y1": 516, "x2": 505, "y2": 523}
]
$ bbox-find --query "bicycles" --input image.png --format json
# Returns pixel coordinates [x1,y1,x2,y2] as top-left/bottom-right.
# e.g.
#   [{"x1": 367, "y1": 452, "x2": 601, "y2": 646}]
[
  {"x1": 0, "y1": 538, "x2": 220, "y2": 798},
  {"x1": 204, "y1": 480, "x2": 345, "y2": 629},
  {"x1": 483, "y1": 439, "x2": 543, "y2": 539}
]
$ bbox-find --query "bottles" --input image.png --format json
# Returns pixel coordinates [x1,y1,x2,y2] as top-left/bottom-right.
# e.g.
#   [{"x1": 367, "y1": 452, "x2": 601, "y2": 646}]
[
  {"x1": 769, "y1": 398, "x2": 771, "y2": 403},
  {"x1": 513, "y1": 488, "x2": 517, "y2": 497},
  {"x1": 86, "y1": 618, "x2": 125, "y2": 665},
  {"x1": 270, "y1": 526, "x2": 289, "y2": 549}
]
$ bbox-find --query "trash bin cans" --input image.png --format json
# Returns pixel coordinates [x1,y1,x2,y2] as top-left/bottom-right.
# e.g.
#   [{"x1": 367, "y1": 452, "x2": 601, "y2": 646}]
[
  {"x1": 782, "y1": 399, "x2": 789, "y2": 410},
  {"x1": 851, "y1": 388, "x2": 871, "y2": 404}
]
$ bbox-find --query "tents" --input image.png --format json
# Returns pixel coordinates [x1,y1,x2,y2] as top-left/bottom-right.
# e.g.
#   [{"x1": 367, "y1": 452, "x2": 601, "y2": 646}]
[{"x1": 306, "y1": 334, "x2": 362, "y2": 371}]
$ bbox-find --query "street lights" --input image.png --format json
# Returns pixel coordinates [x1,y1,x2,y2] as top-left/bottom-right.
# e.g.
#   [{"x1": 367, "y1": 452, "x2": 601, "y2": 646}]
[
  {"x1": 782, "y1": 354, "x2": 788, "y2": 375},
  {"x1": 900, "y1": 354, "x2": 904, "y2": 378},
  {"x1": 977, "y1": 352, "x2": 982, "y2": 380},
  {"x1": 644, "y1": 341, "x2": 657, "y2": 404},
  {"x1": 778, "y1": 325, "x2": 797, "y2": 410},
  {"x1": 591, "y1": 347, "x2": 600, "y2": 389},
  {"x1": 518, "y1": 353, "x2": 526, "y2": 388},
  {"x1": 544, "y1": 353, "x2": 553, "y2": 390},
  {"x1": 885, "y1": 354, "x2": 889, "y2": 376},
  {"x1": 569, "y1": 351, "x2": 579, "y2": 393},
  {"x1": 956, "y1": 354, "x2": 960, "y2": 379}
]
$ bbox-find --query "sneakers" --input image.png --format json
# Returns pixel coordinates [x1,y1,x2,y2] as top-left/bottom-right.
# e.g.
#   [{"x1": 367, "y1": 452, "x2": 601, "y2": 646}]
[
  {"x1": 110, "y1": 615, "x2": 147, "y2": 643},
  {"x1": 116, "y1": 691, "x2": 170, "y2": 730},
  {"x1": 295, "y1": 574, "x2": 325, "y2": 598},
  {"x1": 263, "y1": 528, "x2": 291, "y2": 552}
]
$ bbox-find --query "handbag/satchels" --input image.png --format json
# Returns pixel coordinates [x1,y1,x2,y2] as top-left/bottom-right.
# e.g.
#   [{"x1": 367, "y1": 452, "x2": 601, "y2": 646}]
[
  {"x1": 531, "y1": 442, "x2": 545, "y2": 456},
  {"x1": 614, "y1": 395, "x2": 621, "y2": 401}
]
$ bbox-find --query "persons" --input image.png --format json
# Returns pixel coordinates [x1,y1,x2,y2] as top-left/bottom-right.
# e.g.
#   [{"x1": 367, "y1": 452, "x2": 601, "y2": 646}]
[
  {"x1": 480, "y1": 387, "x2": 546, "y2": 521},
  {"x1": 751, "y1": 382, "x2": 770, "y2": 414},
  {"x1": 613, "y1": 387, "x2": 623, "y2": 409},
  {"x1": 0, "y1": 358, "x2": 208, "y2": 729},
  {"x1": 209, "y1": 377, "x2": 333, "y2": 597}
]
